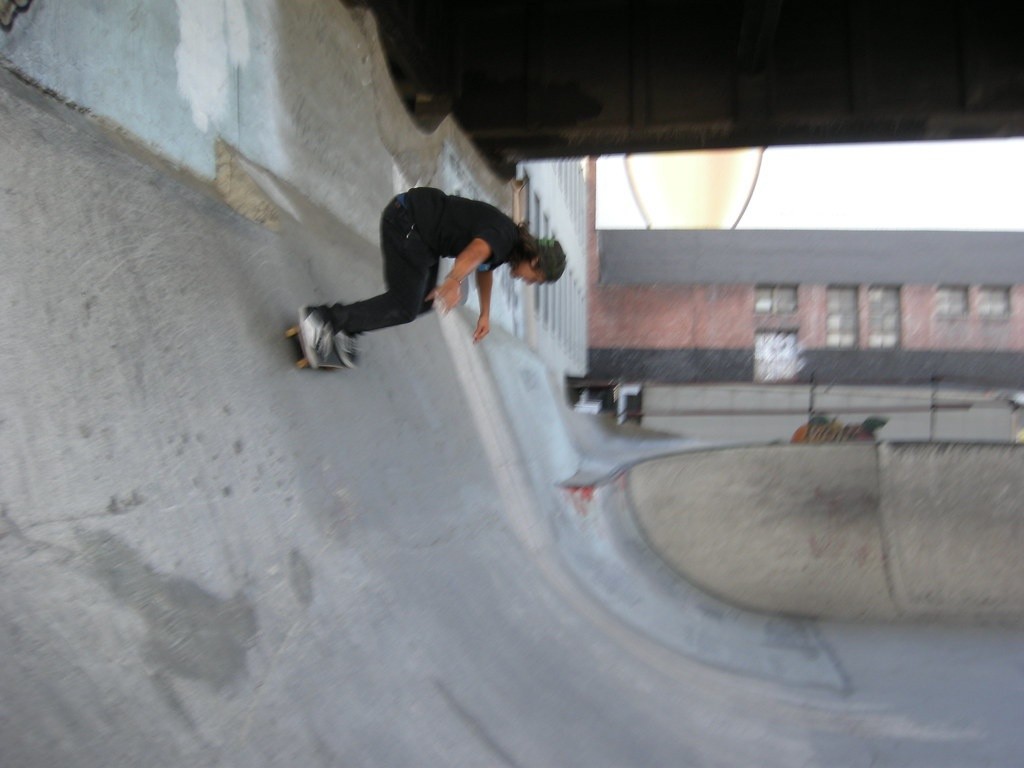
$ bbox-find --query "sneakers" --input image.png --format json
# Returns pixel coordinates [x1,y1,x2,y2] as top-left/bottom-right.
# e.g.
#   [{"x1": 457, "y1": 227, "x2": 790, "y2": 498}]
[
  {"x1": 299, "y1": 305, "x2": 331, "y2": 370},
  {"x1": 333, "y1": 330, "x2": 361, "y2": 369}
]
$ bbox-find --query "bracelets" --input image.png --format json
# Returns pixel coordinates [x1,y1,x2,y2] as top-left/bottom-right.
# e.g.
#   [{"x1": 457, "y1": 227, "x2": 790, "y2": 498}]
[{"x1": 446, "y1": 275, "x2": 462, "y2": 285}]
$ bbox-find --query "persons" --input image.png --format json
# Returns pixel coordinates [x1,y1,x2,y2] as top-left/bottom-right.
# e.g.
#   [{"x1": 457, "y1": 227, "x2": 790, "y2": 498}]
[{"x1": 297, "y1": 187, "x2": 566, "y2": 369}]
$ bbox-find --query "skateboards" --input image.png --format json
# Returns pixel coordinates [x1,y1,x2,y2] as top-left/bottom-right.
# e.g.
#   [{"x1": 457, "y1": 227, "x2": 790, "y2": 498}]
[{"x1": 284, "y1": 303, "x2": 360, "y2": 371}]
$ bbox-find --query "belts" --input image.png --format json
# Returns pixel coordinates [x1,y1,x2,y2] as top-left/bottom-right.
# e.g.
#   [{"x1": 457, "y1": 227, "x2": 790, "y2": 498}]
[{"x1": 393, "y1": 197, "x2": 408, "y2": 216}]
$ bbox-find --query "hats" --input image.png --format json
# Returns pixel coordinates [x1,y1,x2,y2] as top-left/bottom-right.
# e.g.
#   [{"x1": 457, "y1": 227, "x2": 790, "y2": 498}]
[{"x1": 538, "y1": 239, "x2": 566, "y2": 283}]
